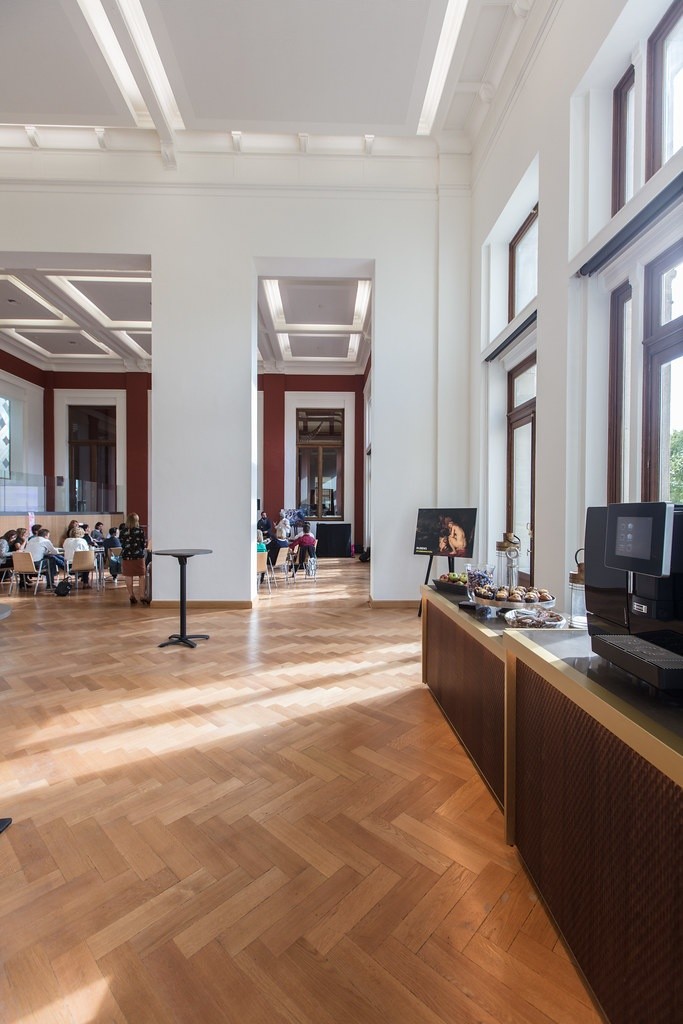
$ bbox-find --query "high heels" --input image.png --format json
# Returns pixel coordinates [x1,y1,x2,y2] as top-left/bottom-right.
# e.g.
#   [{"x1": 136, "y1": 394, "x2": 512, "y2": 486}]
[
  {"x1": 19, "y1": 582, "x2": 33, "y2": 589},
  {"x1": 140, "y1": 597, "x2": 151, "y2": 604},
  {"x1": 130, "y1": 596, "x2": 137, "y2": 604}
]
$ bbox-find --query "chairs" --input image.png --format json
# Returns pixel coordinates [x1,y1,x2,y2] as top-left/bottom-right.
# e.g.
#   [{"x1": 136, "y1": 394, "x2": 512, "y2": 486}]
[
  {"x1": 290, "y1": 546, "x2": 308, "y2": 580},
  {"x1": 271, "y1": 547, "x2": 290, "y2": 585},
  {"x1": 108, "y1": 547, "x2": 122, "y2": 588},
  {"x1": 64, "y1": 551, "x2": 99, "y2": 592},
  {"x1": 8, "y1": 551, "x2": 60, "y2": 597},
  {"x1": 257, "y1": 552, "x2": 272, "y2": 594},
  {"x1": 0, "y1": 567, "x2": 13, "y2": 584}
]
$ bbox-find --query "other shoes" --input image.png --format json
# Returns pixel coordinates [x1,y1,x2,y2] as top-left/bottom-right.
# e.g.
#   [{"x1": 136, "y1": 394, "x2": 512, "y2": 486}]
[
  {"x1": 25, "y1": 578, "x2": 33, "y2": 583},
  {"x1": 83, "y1": 585, "x2": 93, "y2": 590}
]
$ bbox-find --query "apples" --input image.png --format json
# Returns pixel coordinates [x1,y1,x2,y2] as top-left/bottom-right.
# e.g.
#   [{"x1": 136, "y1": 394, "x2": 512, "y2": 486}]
[{"x1": 440, "y1": 573, "x2": 468, "y2": 586}]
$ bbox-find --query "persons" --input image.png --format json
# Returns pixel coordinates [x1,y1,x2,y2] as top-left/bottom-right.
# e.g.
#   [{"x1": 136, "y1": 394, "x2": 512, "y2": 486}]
[
  {"x1": 257, "y1": 529, "x2": 268, "y2": 584},
  {"x1": 27, "y1": 524, "x2": 42, "y2": 541},
  {"x1": 0, "y1": 530, "x2": 33, "y2": 589},
  {"x1": 119, "y1": 512, "x2": 147, "y2": 604},
  {"x1": 265, "y1": 526, "x2": 290, "y2": 566},
  {"x1": 9, "y1": 527, "x2": 33, "y2": 584},
  {"x1": 24, "y1": 528, "x2": 59, "y2": 591},
  {"x1": 63, "y1": 527, "x2": 93, "y2": 589},
  {"x1": 257, "y1": 508, "x2": 315, "y2": 542},
  {"x1": 66, "y1": 520, "x2": 127, "y2": 581},
  {"x1": 288, "y1": 525, "x2": 316, "y2": 577}
]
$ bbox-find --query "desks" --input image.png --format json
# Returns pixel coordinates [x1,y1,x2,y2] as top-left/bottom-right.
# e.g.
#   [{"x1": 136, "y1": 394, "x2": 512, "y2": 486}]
[
  {"x1": 502, "y1": 627, "x2": 683, "y2": 1024},
  {"x1": 49, "y1": 550, "x2": 105, "y2": 588},
  {"x1": 153, "y1": 548, "x2": 213, "y2": 648},
  {"x1": 419, "y1": 583, "x2": 582, "y2": 815}
]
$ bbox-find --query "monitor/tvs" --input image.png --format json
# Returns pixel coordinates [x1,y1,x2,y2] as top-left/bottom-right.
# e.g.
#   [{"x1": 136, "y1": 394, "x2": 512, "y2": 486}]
[{"x1": 604, "y1": 502, "x2": 674, "y2": 578}]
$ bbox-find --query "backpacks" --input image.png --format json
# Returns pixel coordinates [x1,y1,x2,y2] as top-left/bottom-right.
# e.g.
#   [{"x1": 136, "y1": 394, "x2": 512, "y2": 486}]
[{"x1": 54, "y1": 576, "x2": 71, "y2": 596}]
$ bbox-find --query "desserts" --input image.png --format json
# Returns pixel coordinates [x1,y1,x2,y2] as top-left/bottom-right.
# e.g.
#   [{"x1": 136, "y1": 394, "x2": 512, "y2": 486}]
[{"x1": 474, "y1": 584, "x2": 552, "y2": 604}]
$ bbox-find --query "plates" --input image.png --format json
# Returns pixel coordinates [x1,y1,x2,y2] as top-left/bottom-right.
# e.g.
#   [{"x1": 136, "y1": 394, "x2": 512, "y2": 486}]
[{"x1": 504, "y1": 609, "x2": 566, "y2": 628}]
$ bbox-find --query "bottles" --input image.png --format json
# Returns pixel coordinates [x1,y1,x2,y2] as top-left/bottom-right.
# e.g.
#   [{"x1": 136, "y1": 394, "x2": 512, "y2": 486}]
[
  {"x1": 495, "y1": 533, "x2": 521, "y2": 590},
  {"x1": 567, "y1": 550, "x2": 594, "y2": 629}
]
